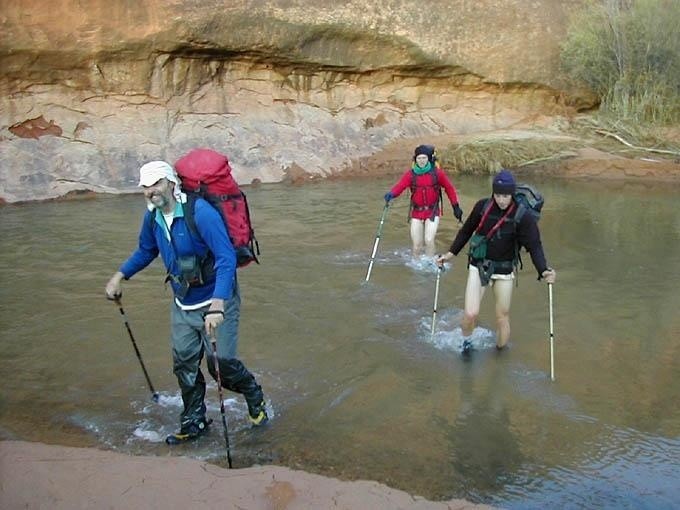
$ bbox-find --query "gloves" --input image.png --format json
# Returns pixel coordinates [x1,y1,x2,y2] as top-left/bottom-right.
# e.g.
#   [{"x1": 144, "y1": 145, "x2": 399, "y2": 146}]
[
  {"x1": 384, "y1": 192, "x2": 395, "y2": 202},
  {"x1": 452, "y1": 203, "x2": 463, "y2": 221}
]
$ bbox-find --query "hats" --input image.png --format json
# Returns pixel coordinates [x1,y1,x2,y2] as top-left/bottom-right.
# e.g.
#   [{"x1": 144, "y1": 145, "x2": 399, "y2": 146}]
[
  {"x1": 415, "y1": 145, "x2": 432, "y2": 155},
  {"x1": 138, "y1": 161, "x2": 188, "y2": 210},
  {"x1": 492, "y1": 169, "x2": 515, "y2": 193}
]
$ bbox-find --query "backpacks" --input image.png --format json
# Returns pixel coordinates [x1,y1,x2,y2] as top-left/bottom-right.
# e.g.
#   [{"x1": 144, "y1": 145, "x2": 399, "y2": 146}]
[
  {"x1": 477, "y1": 190, "x2": 544, "y2": 239},
  {"x1": 409, "y1": 145, "x2": 445, "y2": 209},
  {"x1": 150, "y1": 155, "x2": 260, "y2": 268}
]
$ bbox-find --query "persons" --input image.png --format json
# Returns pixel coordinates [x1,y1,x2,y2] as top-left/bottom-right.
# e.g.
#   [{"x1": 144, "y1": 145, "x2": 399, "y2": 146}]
[
  {"x1": 437, "y1": 170, "x2": 555, "y2": 355},
  {"x1": 104, "y1": 161, "x2": 268, "y2": 443},
  {"x1": 385, "y1": 145, "x2": 463, "y2": 260}
]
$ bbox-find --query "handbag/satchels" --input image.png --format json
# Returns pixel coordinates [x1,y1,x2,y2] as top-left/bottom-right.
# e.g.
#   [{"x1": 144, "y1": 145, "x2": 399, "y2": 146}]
[{"x1": 467, "y1": 233, "x2": 488, "y2": 261}]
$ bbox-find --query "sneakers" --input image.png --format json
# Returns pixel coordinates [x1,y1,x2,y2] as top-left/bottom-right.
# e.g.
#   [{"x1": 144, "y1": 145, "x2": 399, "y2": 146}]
[
  {"x1": 495, "y1": 345, "x2": 506, "y2": 354},
  {"x1": 461, "y1": 340, "x2": 474, "y2": 363},
  {"x1": 166, "y1": 418, "x2": 213, "y2": 445},
  {"x1": 249, "y1": 411, "x2": 269, "y2": 432}
]
